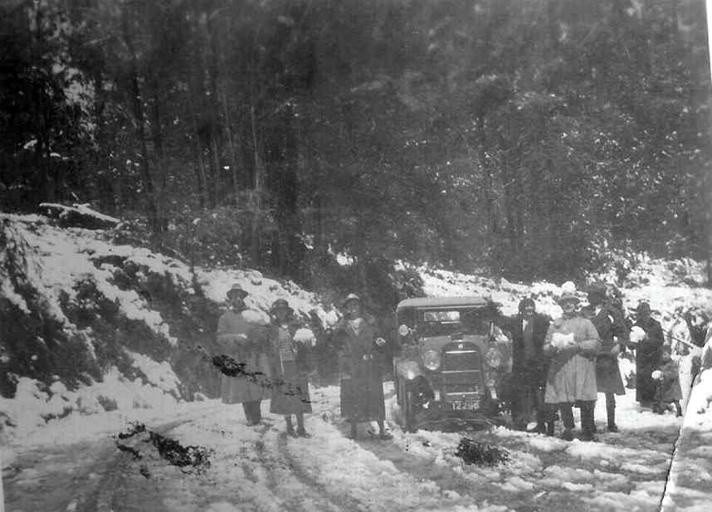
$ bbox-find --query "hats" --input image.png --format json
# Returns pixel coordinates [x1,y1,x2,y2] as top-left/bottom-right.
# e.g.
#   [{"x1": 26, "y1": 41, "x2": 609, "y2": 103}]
[
  {"x1": 270, "y1": 299, "x2": 293, "y2": 314},
  {"x1": 342, "y1": 293, "x2": 361, "y2": 307},
  {"x1": 227, "y1": 282, "x2": 248, "y2": 299},
  {"x1": 558, "y1": 280, "x2": 580, "y2": 305},
  {"x1": 628, "y1": 302, "x2": 655, "y2": 313}
]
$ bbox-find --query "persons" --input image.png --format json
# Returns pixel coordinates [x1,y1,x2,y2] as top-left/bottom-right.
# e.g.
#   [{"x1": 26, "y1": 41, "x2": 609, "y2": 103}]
[
  {"x1": 542, "y1": 291, "x2": 602, "y2": 446},
  {"x1": 487, "y1": 296, "x2": 550, "y2": 434},
  {"x1": 215, "y1": 283, "x2": 267, "y2": 426},
  {"x1": 263, "y1": 299, "x2": 316, "y2": 439},
  {"x1": 627, "y1": 300, "x2": 665, "y2": 408},
  {"x1": 575, "y1": 282, "x2": 629, "y2": 432},
  {"x1": 331, "y1": 293, "x2": 393, "y2": 440},
  {"x1": 653, "y1": 343, "x2": 685, "y2": 418}
]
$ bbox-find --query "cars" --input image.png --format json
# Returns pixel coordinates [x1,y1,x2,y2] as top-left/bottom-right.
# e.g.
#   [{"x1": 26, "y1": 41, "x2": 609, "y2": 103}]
[{"x1": 393, "y1": 297, "x2": 513, "y2": 430}]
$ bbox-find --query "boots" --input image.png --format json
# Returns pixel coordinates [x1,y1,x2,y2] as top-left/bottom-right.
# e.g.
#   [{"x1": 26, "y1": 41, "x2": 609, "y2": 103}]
[
  {"x1": 651, "y1": 404, "x2": 683, "y2": 417},
  {"x1": 287, "y1": 422, "x2": 311, "y2": 438},
  {"x1": 245, "y1": 414, "x2": 261, "y2": 426}
]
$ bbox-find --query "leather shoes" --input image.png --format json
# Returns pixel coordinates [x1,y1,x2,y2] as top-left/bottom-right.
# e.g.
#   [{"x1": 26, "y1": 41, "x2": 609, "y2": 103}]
[{"x1": 529, "y1": 422, "x2": 618, "y2": 441}]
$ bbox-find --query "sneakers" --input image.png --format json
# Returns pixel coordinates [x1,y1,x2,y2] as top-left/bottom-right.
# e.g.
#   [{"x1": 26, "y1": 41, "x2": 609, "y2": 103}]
[
  {"x1": 349, "y1": 430, "x2": 358, "y2": 438},
  {"x1": 378, "y1": 428, "x2": 393, "y2": 438}
]
